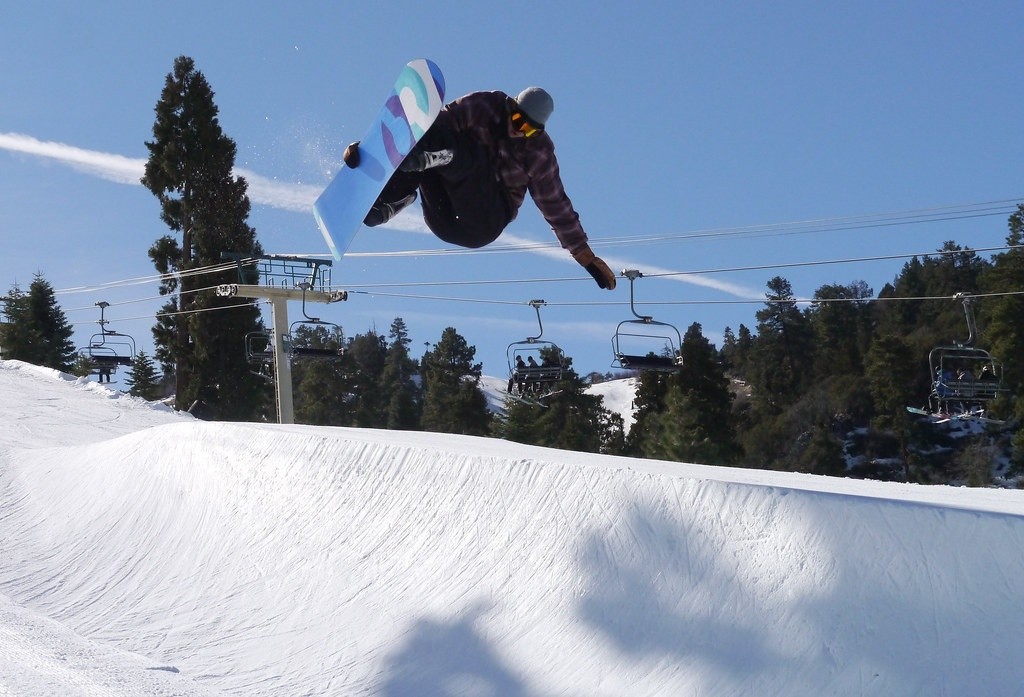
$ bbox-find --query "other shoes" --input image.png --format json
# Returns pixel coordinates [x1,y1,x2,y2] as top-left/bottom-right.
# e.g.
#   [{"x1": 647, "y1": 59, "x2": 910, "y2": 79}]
[
  {"x1": 363, "y1": 207, "x2": 383, "y2": 227},
  {"x1": 400, "y1": 146, "x2": 419, "y2": 172}
]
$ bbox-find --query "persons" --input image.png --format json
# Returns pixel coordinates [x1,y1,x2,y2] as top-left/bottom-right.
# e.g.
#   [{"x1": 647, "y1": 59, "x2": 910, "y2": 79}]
[
  {"x1": 99, "y1": 365, "x2": 110, "y2": 382},
  {"x1": 507, "y1": 355, "x2": 539, "y2": 393},
  {"x1": 344, "y1": 88, "x2": 621, "y2": 289},
  {"x1": 927, "y1": 366, "x2": 996, "y2": 418},
  {"x1": 647, "y1": 351, "x2": 684, "y2": 367}
]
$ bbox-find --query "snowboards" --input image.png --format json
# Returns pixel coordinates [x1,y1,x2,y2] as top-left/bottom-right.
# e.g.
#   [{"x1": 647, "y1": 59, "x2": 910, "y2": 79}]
[
  {"x1": 312, "y1": 58, "x2": 446, "y2": 261},
  {"x1": 99, "y1": 381, "x2": 117, "y2": 384}
]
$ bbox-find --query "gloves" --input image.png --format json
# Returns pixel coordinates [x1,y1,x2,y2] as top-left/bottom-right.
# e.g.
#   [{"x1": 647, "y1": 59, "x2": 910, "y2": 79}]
[
  {"x1": 574, "y1": 243, "x2": 617, "y2": 290},
  {"x1": 343, "y1": 140, "x2": 361, "y2": 169}
]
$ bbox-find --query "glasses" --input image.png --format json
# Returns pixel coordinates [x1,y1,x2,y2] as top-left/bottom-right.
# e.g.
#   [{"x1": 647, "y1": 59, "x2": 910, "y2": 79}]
[{"x1": 511, "y1": 104, "x2": 544, "y2": 139}]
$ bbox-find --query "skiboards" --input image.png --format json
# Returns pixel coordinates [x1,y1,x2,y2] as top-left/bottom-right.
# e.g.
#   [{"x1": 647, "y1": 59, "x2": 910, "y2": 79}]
[
  {"x1": 495, "y1": 379, "x2": 548, "y2": 408},
  {"x1": 538, "y1": 390, "x2": 564, "y2": 399},
  {"x1": 906, "y1": 406, "x2": 1005, "y2": 425}
]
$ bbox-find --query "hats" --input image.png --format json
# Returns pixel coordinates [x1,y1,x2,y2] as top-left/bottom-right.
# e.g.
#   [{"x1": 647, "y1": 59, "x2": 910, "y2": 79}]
[
  {"x1": 983, "y1": 366, "x2": 990, "y2": 371},
  {"x1": 517, "y1": 86, "x2": 554, "y2": 125}
]
